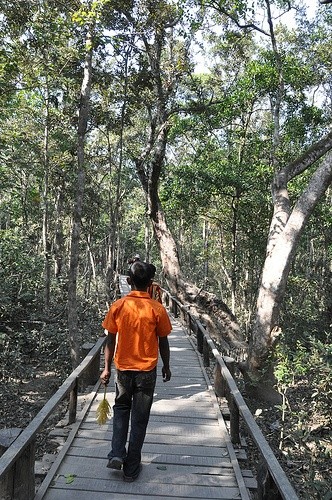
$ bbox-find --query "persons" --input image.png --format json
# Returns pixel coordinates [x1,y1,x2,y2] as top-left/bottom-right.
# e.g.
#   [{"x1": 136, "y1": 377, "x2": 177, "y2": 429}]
[
  {"x1": 147, "y1": 283, "x2": 163, "y2": 305},
  {"x1": 126, "y1": 254, "x2": 140, "y2": 290},
  {"x1": 99, "y1": 261, "x2": 173, "y2": 481}
]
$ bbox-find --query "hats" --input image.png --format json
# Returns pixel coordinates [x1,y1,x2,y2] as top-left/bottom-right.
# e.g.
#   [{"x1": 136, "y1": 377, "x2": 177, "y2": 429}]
[{"x1": 128, "y1": 260, "x2": 156, "y2": 284}]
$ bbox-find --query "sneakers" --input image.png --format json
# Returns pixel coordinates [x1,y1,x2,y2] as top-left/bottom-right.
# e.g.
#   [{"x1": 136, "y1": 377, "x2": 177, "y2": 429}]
[
  {"x1": 106, "y1": 457, "x2": 123, "y2": 470},
  {"x1": 123, "y1": 463, "x2": 142, "y2": 481}
]
